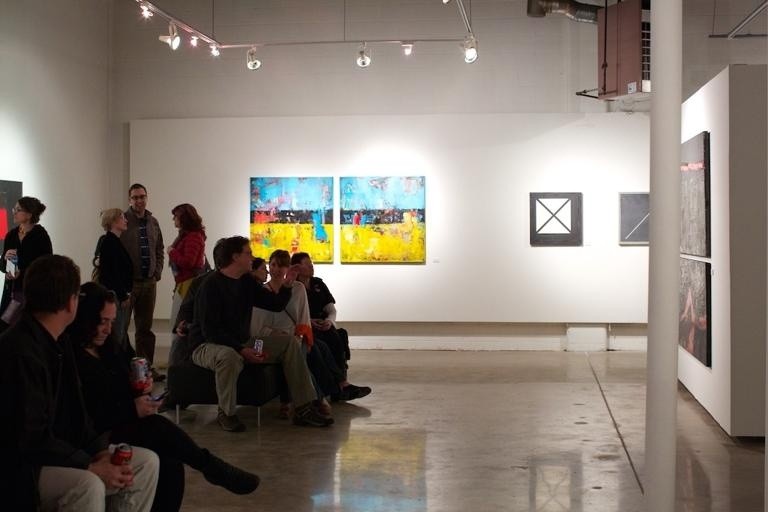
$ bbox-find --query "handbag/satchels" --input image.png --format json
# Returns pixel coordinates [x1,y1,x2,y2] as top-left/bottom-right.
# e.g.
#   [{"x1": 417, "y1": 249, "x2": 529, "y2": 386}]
[
  {"x1": 1, "y1": 299, "x2": 21, "y2": 324},
  {"x1": 192, "y1": 255, "x2": 211, "y2": 276},
  {"x1": 164, "y1": 385, "x2": 192, "y2": 409},
  {"x1": 91, "y1": 266, "x2": 99, "y2": 283},
  {"x1": 5, "y1": 259, "x2": 19, "y2": 277},
  {"x1": 169, "y1": 329, "x2": 198, "y2": 370}
]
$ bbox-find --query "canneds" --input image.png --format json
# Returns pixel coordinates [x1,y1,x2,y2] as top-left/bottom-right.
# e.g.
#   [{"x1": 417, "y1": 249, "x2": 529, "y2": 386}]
[
  {"x1": 113, "y1": 443, "x2": 135, "y2": 488},
  {"x1": 128, "y1": 356, "x2": 153, "y2": 395},
  {"x1": 253, "y1": 338, "x2": 264, "y2": 357}
]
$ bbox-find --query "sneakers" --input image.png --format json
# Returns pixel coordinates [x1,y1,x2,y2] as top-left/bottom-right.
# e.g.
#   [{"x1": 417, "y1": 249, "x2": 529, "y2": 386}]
[
  {"x1": 278, "y1": 397, "x2": 334, "y2": 427},
  {"x1": 218, "y1": 406, "x2": 246, "y2": 432},
  {"x1": 329, "y1": 382, "x2": 371, "y2": 402},
  {"x1": 150, "y1": 368, "x2": 166, "y2": 382},
  {"x1": 202, "y1": 454, "x2": 259, "y2": 495}
]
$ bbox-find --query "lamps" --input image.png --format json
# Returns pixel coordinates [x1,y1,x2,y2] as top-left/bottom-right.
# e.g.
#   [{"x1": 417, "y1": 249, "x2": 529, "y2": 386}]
[{"x1": 121, "y1": 0, "x2": 481, "y2": 73}]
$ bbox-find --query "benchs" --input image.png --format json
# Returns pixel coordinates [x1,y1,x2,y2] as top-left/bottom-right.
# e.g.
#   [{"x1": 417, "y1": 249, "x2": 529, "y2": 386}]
[{"x1": 166, "y1": 361, "x2": 284, "y2": 429}]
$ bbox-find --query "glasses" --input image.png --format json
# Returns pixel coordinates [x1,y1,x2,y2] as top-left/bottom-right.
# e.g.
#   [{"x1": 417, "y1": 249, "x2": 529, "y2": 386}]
[
  {"x1": 11, "y1": 208, "x2": 26, "y2": 215},
  {"x1": 129, "y1": 195, "x2": 146, "y2": 200}
]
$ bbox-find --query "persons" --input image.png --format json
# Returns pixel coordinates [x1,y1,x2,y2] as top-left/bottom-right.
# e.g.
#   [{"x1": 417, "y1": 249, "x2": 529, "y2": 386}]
[
  {"x1": 91, "y1": 208, "x2": 139, "y2": 369},
  {"x1": 0, "y1": 196, "x2": 53, "y2": 332},
  {"x1": 167, "y1": 203, "x2": 212, "y2": 348},
  {"x1": 61, "y1": 280, "x2": 260, "y2": 512},
  {"x1": 155, "y1": 234, "x2": 373, "y2": 431},
  {"x1": 120, "y1": 183, "x2": 168, "y2": 387},
  {"x1": 0, "y1": 253, "x2": 161, "y2": 512}
]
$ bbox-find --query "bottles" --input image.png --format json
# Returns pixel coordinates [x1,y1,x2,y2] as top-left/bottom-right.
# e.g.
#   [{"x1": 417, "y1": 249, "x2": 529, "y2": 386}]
[{"x1": 10, "y1": 253, "x2": 18, "y2": 271}]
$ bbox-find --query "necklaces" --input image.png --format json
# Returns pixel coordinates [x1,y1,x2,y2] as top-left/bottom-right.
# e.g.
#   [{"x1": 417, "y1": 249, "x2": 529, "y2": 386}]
[{"x1": 18, "y1": 228, "x2": 28, "y2": 235}]
